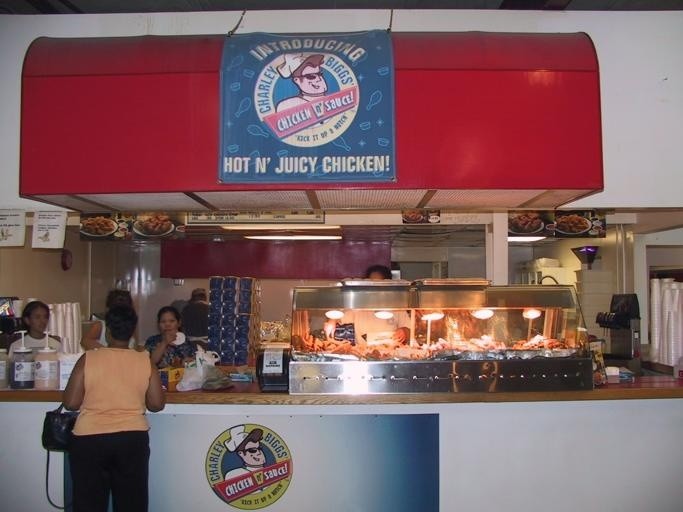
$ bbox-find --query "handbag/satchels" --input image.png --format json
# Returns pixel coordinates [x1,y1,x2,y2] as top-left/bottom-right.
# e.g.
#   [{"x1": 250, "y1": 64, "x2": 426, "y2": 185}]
[
  {"x1": 42, "y1": 403, "x2": 80, "y2": 451},
  {"x1": 204, "y1": 365, "x2": 234, "y2": 390}
]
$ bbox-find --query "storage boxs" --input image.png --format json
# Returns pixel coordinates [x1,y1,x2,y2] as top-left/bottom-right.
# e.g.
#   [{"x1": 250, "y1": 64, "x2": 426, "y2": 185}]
[{"x1": 574, "y1": 270, "x2": 609, "y2": 341}]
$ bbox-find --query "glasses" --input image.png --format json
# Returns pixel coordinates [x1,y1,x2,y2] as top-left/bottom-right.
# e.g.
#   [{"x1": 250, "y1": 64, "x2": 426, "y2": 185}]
[
  {"x1": 298, "y1": 72, "x2": 322, "y2": 79},
  {"x1": 245, "y1": 444, "x2": 263, "y2": 453}
]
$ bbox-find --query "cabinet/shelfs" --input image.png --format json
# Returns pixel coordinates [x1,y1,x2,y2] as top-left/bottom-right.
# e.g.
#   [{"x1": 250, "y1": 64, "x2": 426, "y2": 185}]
[
  {"x1": 289, "y1": 285, "x2": 594, "y2": 395},
  {"x1": 517, "y1": 268, "x2": 564, "y2": 284}
]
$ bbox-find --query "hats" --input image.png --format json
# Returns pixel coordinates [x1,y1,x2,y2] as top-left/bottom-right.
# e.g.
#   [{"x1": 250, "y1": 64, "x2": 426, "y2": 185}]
[
  {"x1": 187, "y1": 288, "x2": 206, "y2": 301},
  {"x1": 224, "y1": 426, "x2": 263, "y2": 452},
  {"x1": 277, "y1": 52, "x2": 323, "y2": 78}
]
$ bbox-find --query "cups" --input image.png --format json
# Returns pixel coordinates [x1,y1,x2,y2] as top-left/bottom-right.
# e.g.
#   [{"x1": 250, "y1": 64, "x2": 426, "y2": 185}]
[{"x1": 12, "y1": 297, "x2": 81, "y2": 354}]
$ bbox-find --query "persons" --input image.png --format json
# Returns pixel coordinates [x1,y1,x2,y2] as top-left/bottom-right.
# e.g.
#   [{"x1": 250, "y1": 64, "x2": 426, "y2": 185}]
[
  {"x1": 145, "y1": 306, "x2": 198, "y2": 369},
  {"x1": 179, "y1": 288, "x2": 210, "y2": 350},
  {"x1": 8, "y1": 301, "x2": 64, "y2": 360},
  {"x1": 80, "y1": 288, "x2": 138, "y2": 350},
  {"x1": 64, "y1": 302, "x2": 165, "y2": 512},
  {"x1": 324, "y1": 265, "x2": 411, "y2": 348}
]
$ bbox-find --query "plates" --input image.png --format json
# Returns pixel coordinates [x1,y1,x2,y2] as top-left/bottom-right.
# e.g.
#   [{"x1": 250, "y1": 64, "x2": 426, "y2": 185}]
[
  {"x1": 546, "y1": 224, "x2": 557, "y2": 231},
  {"x1": 176, "y1": 226, "x2": 186, "y2": 233},
  {"x1": 132, "y1": 219, "x2": 174, "y2": 237},
  {"x1": 508, "y1": 222, "x2": 545, "y2": 234},
  {"x1": 115, "y1": 218, "x2": 129, "y2": 240},
  {"x1": 553, "y1": 218, "x2": 592, "y2": 235},
  {"x1": 403, "y1": 216, "x2": 424, "y2": 223},
  {"x1": 80, "y1": 220, "x2": 118, "y2": 236},
  {"x1": 428, "y1": 216, "x2": 440, "y2": 223},
  {"x1": 588, "y1": 217, "x2": 603, "y2": 236}
]
